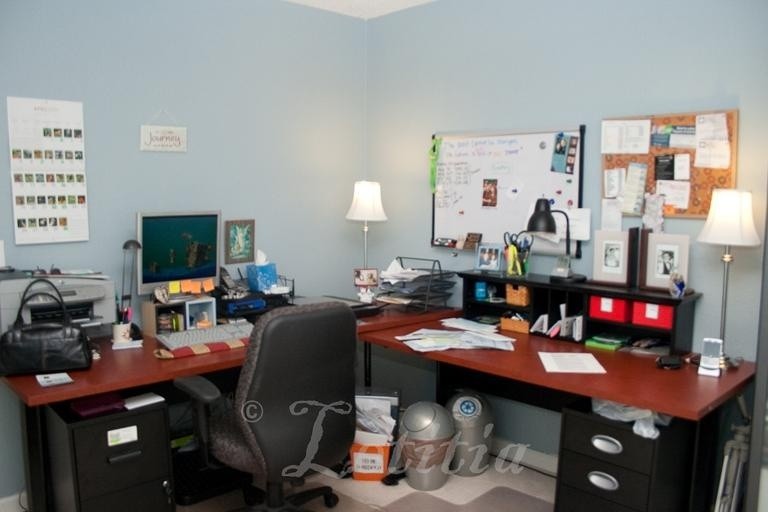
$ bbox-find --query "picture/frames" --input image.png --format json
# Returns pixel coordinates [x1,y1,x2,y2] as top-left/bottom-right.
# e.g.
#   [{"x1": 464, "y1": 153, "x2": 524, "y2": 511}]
[
  {"x1": 224, "y1": 219, "x2": 254, "y2": 265},
  {"x1": 645, "y1": 233, "x2": 690, "y2": 291},
  {"x1": 593, "y1": 231, "x2": 629, "y2": 283},
  {"x1": 601, "y1": 109, "x2": 739, "y2": 219}
]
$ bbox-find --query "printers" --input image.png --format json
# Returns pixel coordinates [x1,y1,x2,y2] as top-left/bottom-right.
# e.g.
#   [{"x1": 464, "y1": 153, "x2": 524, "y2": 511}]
[{"x1": 0, "y1": 270, "x2": 118, "y2": 340}]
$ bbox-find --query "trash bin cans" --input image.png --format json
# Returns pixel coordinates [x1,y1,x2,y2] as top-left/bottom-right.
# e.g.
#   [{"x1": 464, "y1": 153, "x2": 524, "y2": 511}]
[
  {"x1": 446, "y1": 392, "x2": 493, "y2": 477},
  {"x1": 397, "y1": 400, "x2": 454, "y2": 491}
]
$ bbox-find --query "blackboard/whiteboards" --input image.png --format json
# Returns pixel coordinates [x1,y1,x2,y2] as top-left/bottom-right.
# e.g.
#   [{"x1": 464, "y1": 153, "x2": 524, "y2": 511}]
[{"x1": 430, "y1": 124, "x2": 585, "y2": 259}]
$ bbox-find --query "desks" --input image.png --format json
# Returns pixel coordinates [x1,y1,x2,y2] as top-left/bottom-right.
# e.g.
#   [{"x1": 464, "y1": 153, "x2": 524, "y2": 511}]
[
  {"x1": 357, "y1": 316, "x2": 756, "y2": 511},
  {"x1": 1, "y1": 295, "x2": 459, "y2": 511}
]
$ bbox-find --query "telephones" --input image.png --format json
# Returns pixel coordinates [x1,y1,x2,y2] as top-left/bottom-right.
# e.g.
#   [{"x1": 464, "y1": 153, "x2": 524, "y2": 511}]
[{"x1": 218, "y1": 267, "x2": 245, "y2": 295}]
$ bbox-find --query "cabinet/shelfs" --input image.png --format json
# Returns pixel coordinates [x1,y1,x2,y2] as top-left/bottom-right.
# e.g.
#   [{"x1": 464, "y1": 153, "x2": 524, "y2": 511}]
[{"x1": 456, "y1": 268, "x2": 703, "y2": 356}]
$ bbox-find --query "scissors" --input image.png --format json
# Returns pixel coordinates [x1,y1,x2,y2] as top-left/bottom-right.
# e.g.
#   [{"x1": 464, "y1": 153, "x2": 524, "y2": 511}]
[{"x1": 504, "y1": 231, "x2": 534, "y2": 252}]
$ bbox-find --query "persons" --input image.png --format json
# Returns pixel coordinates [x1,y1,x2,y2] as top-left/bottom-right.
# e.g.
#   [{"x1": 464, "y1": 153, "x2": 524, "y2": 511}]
[
  {"x1": 555, "y1": 132, "x2": 569, "y2": 154},
  {"x1": 605, "y1": 244, "x2": 621, "y2": 270},
  {"x1": 480, "y1": 248, "x2": 498, "y2": 266},
  {"x1": 482, "y1": 178, "x2": 498, "y2": 206},
  {"x1": 654, "y1": 250, "x2": 675, "y2": 277}
]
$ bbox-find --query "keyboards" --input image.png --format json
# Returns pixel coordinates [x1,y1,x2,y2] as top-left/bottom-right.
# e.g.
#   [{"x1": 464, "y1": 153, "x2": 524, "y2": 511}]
[{"x1": 154, "y1": 320, "x2": 255, "y2": 351}]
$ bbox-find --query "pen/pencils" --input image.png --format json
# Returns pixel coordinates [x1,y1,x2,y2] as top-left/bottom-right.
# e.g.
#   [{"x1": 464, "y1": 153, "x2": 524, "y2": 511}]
[
  {"x1": 116, "y1": 295, "x2": 133, "y2": 324},
  {"x1": 511, "y1": 237, "x2": 530, "y2": 274}
]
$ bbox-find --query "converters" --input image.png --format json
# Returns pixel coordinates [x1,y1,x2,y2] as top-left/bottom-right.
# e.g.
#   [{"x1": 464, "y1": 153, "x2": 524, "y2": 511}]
[{"x1": 656, "y1": 355, "x2": 682, "y2": 369}]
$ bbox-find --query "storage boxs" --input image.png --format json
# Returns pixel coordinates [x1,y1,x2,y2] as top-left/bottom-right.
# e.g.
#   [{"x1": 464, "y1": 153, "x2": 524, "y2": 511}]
[
  {"x1": 631, "y1": 300, "x2": 675, "y2": 330},
  {"x1": 588, "y1": 295, "x2": 631, "y2": 323},
  {"x1": 350, "y1": 443, "x2": 395, "y2": 481}
]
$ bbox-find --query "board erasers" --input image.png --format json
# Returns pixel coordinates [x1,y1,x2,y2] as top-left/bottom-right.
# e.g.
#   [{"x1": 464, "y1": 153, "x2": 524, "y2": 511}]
[{"x1": 463, "y1": 233, "x2": 482, "y2": 249}]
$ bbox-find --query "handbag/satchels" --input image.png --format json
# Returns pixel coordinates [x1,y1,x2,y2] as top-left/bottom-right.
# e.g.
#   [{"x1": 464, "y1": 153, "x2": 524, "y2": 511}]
[{"x1": 0, "y1": 278, "x2": 94, "y2": 378}]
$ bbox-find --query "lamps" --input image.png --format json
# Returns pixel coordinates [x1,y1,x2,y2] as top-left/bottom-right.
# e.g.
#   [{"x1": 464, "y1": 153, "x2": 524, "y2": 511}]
[
  {"x1": 477, "y1": 245, "x2": 501, "y2": 271},
  {"x1": 695, "y1": 188, "x2": 761, "y2": 370},
  {"x1": 526, "y1": 199, "x2": 574, "y2": 278},
  {"x1": 345, "y1": 180, "x2": 389, "y2": 304}
]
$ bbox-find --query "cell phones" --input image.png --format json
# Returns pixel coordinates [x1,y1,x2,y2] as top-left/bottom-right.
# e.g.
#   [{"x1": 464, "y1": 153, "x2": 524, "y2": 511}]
[{"x1": 699, "y1": 337, "x2": 723, "y2": 369}]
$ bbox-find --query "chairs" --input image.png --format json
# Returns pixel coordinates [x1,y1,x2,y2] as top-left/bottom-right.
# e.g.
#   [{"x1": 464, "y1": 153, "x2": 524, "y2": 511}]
[{"x1": 172, "y1": 302, "x2": 356, "y2": 512}]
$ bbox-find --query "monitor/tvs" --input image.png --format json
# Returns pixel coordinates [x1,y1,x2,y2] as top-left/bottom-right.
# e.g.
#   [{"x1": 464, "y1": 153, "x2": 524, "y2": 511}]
[{"x1": 134, "y1": 209, "x2": 222, "y2": 300}]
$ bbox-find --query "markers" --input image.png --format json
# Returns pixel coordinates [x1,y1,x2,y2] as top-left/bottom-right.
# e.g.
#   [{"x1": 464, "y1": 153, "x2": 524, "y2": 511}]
[{"x1": 434, "y1": 238, "x2": 457, "y2": 247}]
